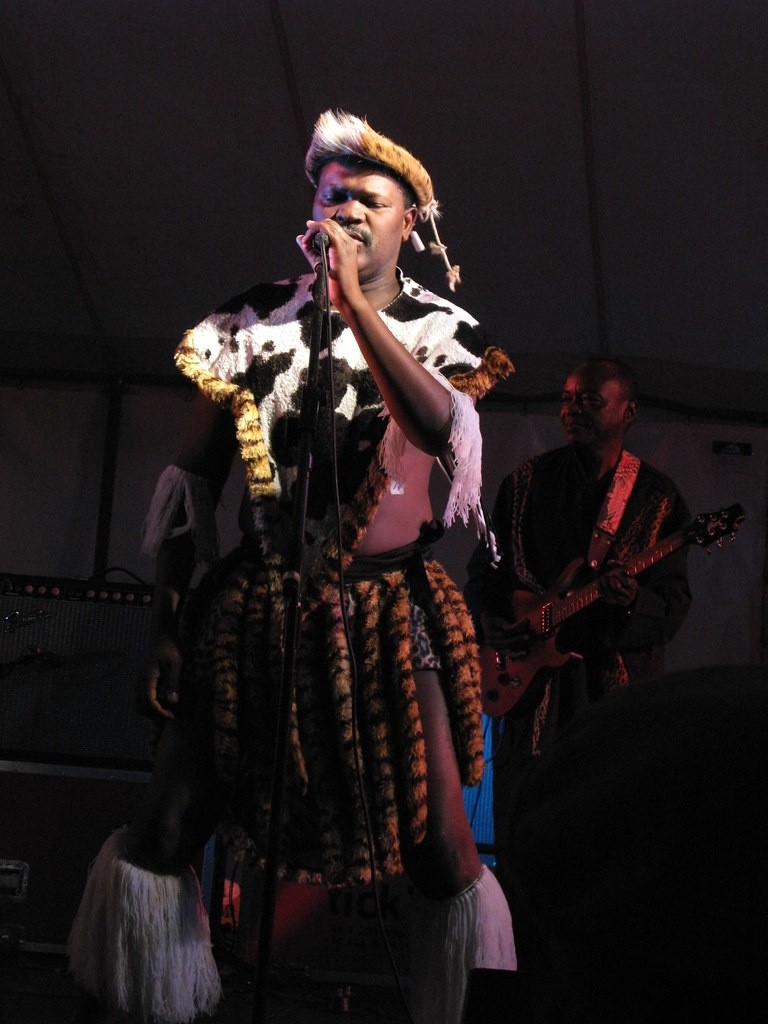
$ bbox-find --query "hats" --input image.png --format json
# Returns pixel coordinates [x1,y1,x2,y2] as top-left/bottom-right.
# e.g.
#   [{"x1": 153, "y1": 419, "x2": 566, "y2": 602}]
[{"x1": 302, "y1": 110, "x2": 465, "y2": 291}]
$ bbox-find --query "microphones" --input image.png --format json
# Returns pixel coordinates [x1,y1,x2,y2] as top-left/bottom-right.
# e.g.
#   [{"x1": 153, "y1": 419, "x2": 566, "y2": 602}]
[
  {"x1": 313, "y1": 232, "x2": 332, "y2": 255},
  {"x1": 27, "y1": 644, "x2": 61, "y2": 669}
]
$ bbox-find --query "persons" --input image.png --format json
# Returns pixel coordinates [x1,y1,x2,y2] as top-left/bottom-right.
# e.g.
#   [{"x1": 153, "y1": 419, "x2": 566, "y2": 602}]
[
  {"x1": 66, "y1": 109, "x2": 518, "y2": 1024},
  {"x1": 461, "y1": 354, "x2": 693, "y2": 764}
]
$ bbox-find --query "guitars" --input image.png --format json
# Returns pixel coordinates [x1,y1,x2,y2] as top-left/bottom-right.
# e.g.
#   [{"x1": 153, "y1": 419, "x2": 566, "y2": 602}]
[{"x1": 468, "y1": 495, "x2": 747, "y2": 718}]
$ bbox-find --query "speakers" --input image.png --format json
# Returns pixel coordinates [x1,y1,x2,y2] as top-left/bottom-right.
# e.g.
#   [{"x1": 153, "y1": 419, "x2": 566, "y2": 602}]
[{"x1": 0, "y1": 573, "x2": 183, "y2": 772}]
